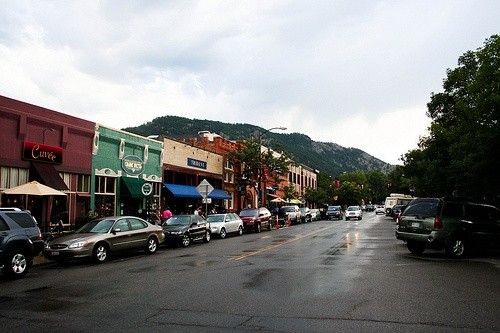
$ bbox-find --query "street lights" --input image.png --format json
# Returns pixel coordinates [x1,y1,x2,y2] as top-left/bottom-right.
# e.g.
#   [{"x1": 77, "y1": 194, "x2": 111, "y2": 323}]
[{"x1": 260, "y1": 127, "x2": 288, "y2": 206}]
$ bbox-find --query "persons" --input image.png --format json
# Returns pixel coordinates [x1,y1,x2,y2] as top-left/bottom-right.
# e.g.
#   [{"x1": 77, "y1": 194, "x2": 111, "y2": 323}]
[{"x1": 156, "y1": 206, "x2": 226, "y2": 220}]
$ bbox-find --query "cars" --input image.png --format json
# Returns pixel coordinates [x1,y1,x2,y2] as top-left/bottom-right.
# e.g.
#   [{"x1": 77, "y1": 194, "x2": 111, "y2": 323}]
[
  {"x1": 43, "y1": 216, "x2": 166, "y2": 264},
  {"x1": 238, "y1": 206, "x2": 272, "y2": 233},
  {"x1": 360, "y1": 204, "x2": 384, "y2": 214},
  {"x1": 325, "y1": 206, "x2": 343, "y2": 220},
  {"x1": 202, "y1": 212, "x2": 244, "y2": 238},
  {"x1": 392, "y1": 205, "x2": 406, "y2": 220},
  {"x1": 344, "y1": 206, "x2": 363, "y2": 220},
  {"x1": 0, "y1": 206, "x2": 44, "y2": 277},
  {"x1": 280, "y1": 205, "x2": 301, "y2": 224},
  {"x1": 384, "y1": 193, "x2": 419, "y2": 218},
  {"x1": 159, "y1": 215, "x2": 212, "y2": 247},
  {"x1": 300, "y1": 207, "x2": 313, "y2": 223},
  {"x1": 394, "y1": 198, "x2": 500, "y2": 258},
  {"x1": 310, "y1": 209, "x2": 322, "y2": 220}
]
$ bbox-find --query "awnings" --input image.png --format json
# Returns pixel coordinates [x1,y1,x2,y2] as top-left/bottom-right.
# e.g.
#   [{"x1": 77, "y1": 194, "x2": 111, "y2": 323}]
[
  {"x1": 30, "y1": 161, "x2": 70, "y2": 190},
  {"x1": 268, "y1": 194, "x2": 306, "y2": 204},
  {"x1": 121, "y1": 176, "x2": 153, "y2": 198},
  {"x1": 163, "y1": 184, "x2": 232, "y2": 199}
]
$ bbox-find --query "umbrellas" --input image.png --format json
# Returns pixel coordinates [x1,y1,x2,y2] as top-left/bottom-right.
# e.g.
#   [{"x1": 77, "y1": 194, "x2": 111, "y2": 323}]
[{"x1": 1, "y1": 180, "x2": 68, "y2": 196}]
